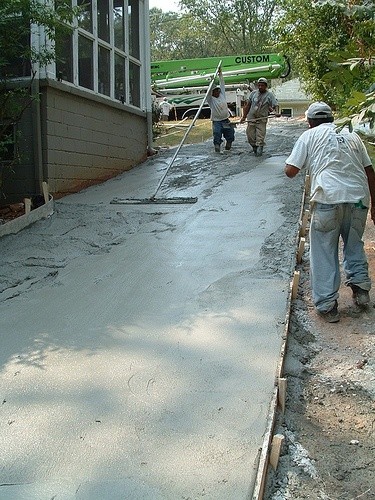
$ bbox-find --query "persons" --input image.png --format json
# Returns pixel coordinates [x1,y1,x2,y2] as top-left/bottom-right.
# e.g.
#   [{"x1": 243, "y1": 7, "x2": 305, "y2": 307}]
[
  {"x1": 240, "y1": 78, "x2": 277, "y2": 156},
  {"x1": 206, "y1": 65, "x2": 235, "y2": 152},
  {"x1": 285, "y1": 101, "x2": 375, "y2": 323},
  {"x1": 159, "y1": 97, "x2": 172, "y2": 121}
]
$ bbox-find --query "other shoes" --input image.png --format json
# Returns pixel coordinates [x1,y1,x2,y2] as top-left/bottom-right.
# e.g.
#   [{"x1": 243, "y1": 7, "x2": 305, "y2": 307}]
[
  {"x1": 317, "y1": 300, "x2": 339, "y2": 322},
  {"x1": 347, "y1": 283, "x2": 370, "y2": 305}
]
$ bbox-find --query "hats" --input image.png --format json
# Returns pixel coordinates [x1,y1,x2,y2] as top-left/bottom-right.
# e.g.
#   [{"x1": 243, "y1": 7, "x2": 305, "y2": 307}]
[
  {"x1": 212, "y1": 84, "x2": 220, "y2": 90},
  {"x1": 258, "y1": 77, "x2": 267, "y2": 83},
  {"x1": 305, "y1": 101, "x2": 333, "y2": 118}
]
